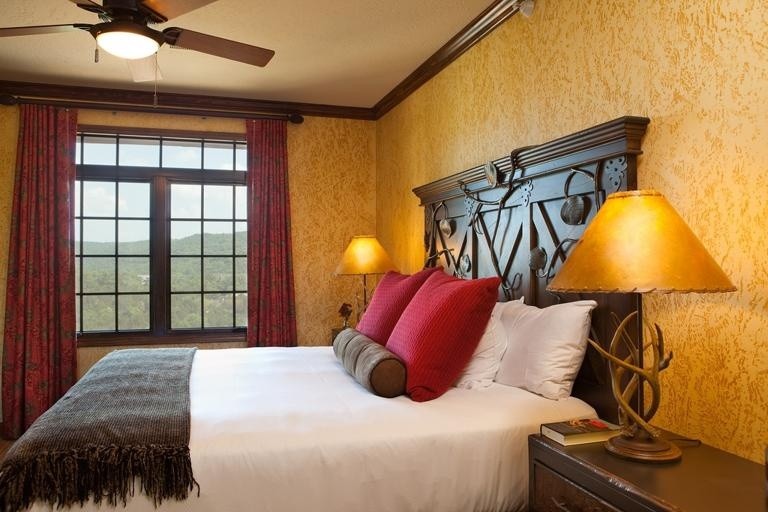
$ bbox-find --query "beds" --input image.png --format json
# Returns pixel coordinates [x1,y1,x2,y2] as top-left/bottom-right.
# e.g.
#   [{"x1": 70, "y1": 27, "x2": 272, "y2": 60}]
[{"x1": 3, "y1": 116, "x2": 652, "y2": 511}]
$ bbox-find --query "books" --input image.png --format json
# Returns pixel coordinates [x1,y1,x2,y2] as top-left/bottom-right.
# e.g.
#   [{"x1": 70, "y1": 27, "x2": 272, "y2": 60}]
[{"x1": 540, "y1": 415, "x2": 620, "y2": 446}]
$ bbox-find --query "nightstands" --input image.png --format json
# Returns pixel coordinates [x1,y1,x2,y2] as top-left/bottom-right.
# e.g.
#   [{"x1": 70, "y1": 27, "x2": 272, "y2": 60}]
[
  {"x1": 331, "y1": 325, "x2": 355, "y2": 346},
  {"x1": 527, "y1": 423, "x2": 768, "y2": 512}
]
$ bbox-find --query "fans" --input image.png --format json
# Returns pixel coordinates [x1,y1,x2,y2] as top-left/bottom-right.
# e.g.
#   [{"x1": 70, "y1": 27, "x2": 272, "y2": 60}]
[{"x1": 0, "y1": 1, "x2": 273, "y2": 66}]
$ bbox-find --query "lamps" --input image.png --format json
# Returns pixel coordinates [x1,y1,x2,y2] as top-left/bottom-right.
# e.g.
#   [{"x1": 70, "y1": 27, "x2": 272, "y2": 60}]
[
  {"x1": 336, "y1": 236, "x2": 399, "y2": 342},
  {"x1": 543, "y1": 193, "x2": 736, "y2": 465},
  {"x1": 95, "y1": 29, "x2": 158, "y2": 60}
]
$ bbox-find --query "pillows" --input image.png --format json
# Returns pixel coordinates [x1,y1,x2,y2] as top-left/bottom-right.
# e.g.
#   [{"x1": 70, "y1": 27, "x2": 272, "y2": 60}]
[
  {"x1": 353, "y1": 267, "x2": 445, "y2": 346},
  {"x1": 334, "y1": 328, "x2": 407, "y2": 397},
  {"x1": 453, "y1": 307, "x2": 507, "y2": 392},
  {"x1": 381, "y1": 270, "x2": 501, "y2": 402},
  {"x1": 494, "y1": 300, "x2": 598, "y2": 401}
]
